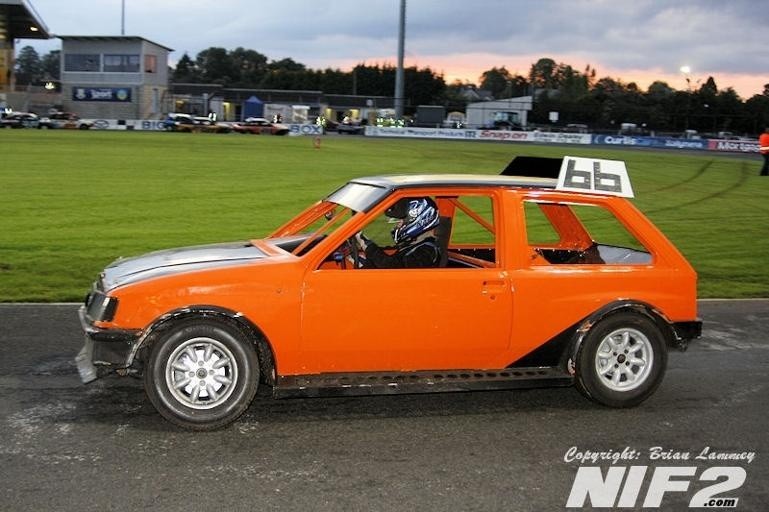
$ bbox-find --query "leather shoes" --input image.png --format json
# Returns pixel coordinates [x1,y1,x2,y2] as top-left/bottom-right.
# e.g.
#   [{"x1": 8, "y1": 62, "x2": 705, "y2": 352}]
[{"x1": 354, "y1": 230, "x2": 374, "y2": 252}]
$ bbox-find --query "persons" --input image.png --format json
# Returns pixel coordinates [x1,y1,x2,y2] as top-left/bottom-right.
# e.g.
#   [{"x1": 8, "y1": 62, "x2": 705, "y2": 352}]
[
  {"x1": 355, "y1": 196, "x2": 443, "y2": 268},
  {"x1": 758, "y1": 128, "x2": 769, "y2": 176}
]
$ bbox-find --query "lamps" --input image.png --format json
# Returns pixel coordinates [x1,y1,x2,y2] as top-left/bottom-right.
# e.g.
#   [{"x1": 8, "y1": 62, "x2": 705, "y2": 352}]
[{"x1": 431, "y1": 215, "x2": 452, "y2": 268}]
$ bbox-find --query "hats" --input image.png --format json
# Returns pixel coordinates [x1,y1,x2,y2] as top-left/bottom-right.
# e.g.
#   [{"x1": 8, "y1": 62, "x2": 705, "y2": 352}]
[
  {"x1": 78, "y1": 156, "x2": 703, "y2": 431},
  {"x1": 717, "y1": 131, "x2": 739, "y2": 140},
  {"x1": 684, "y1": 130, "x2": 701, "y2": 140},
  {"x1": 0, "y1": 111, "x2": 95, "y2": 129},
  {"x1": 477, "y1": 120, "x2": 526, "y2": 131},
  {"x1": 163, "y1": 113, "x2": 290, "y2": 135}
]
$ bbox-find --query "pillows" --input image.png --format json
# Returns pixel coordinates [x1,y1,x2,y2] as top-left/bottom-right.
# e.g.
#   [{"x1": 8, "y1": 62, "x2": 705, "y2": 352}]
[{"x1": 384, "y1": 195, "x2": 442, "y2": 246}]
